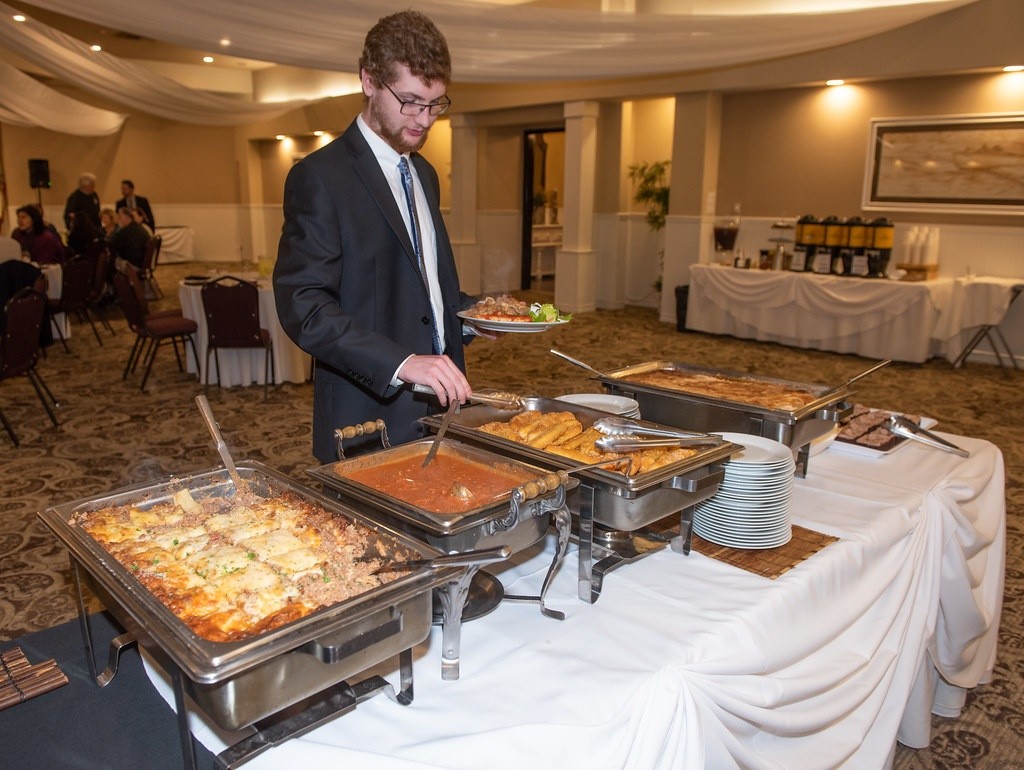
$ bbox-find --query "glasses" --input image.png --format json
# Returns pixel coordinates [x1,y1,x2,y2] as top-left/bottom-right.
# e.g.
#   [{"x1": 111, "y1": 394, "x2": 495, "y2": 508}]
[{"x1": 372, "y1": 70, "x2": 452, "y2": 116}]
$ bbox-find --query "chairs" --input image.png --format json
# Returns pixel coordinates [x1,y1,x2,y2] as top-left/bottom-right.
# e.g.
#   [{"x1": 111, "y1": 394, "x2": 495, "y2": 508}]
[
  {"x1": 0, "y1": 234, "x2": 201, "y2": 449},
  {"x1": 201, "y1": 274, "x2": 275, "y2": 401}
]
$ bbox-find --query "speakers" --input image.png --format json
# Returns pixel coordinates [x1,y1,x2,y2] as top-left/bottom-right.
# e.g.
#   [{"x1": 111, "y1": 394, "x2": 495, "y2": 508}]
[{"x1": 28, "y1": 159, "x2": 51, "y2": 190}]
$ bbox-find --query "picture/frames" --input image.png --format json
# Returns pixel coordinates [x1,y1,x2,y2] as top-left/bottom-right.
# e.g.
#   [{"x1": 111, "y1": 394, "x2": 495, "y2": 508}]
[{"x1": 860, "y1": 112, "x2": 1024, "y2": 216}]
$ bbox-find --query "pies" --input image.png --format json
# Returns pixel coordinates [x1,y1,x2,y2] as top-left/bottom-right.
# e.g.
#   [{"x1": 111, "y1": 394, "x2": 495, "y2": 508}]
[{"x1": 620, "y1": 368, "x2": 819, "y2": 412}]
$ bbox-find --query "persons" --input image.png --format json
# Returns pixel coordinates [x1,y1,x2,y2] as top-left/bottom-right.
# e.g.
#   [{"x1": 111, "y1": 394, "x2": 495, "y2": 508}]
[
  {"x1": 10, "y1": 203, "x2": 69, "y2": 265},
  {"x1": 67, "y1": 206, "x2": 154, "y2": 305},
  {"x1": 0, "y1": 193, "x2": 21, "y2": 262},
  {"x1": 272, "y1": 8, "x2": 507, "y2": 506},
  {"x1": 64, "y1": 171, "x2": 101, "y2": 238},
  {"x1": 114, "y1": 180, "x2": 155, "y2": 235}
]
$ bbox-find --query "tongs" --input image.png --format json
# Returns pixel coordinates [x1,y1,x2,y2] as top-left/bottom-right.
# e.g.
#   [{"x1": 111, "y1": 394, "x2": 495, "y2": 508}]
[
  {"x1": 881, "y1": 413, "x2": 971, "y2": 458},
  {"x1": 412, "y1": 383, "x2": 526, "y2": 412},
  {"x1": 593, "y1": 416, "x2": 722, "y2": 453}
]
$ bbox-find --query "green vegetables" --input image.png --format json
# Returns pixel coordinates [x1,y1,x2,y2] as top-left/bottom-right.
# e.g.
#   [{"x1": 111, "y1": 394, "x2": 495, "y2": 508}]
[
  {"x1": 528, "y1": 302, "x2": 574, "y2": 322},
  {"x1": 131, "y1": 539, "x2": 332, "y2": 583}
]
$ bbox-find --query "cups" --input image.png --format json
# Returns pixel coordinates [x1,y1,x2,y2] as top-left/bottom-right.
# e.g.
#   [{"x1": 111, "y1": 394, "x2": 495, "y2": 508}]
[
  {"x1": 900, "y1": 224, "x2": 939, "y2": 266},
  {"x1": 258, "y1": 254, "x2": 276, "y2": 279}
]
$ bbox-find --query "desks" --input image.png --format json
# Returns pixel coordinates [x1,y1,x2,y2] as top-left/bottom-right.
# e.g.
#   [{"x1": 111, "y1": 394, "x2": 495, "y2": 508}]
[
  {"x1": 177, "y1": 273, "x2": 312, "y2": 387},
  {"x1": 685, "y1": 263, "x2": 1024, "y2": 382},
  {"x1": 156, "y1": 225, "x2": 195, "y2": 264},
  {"x1": 0, "y1": 429, "x2": 1003, "y2": 770},
  {"x1": 39, "y1": 261, "x2": 71, "y2": 340}
]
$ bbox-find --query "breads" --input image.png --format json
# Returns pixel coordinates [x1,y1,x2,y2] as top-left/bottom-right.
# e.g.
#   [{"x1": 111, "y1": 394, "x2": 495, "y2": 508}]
[{"x1": 479, "y1": 410, "x2": 697, "y2": 476}]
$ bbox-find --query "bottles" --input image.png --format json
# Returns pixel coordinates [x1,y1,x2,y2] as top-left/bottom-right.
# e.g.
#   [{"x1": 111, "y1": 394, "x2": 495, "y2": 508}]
[{"x1": 759, "y1": 241, "x2": 786, "y2": 271}]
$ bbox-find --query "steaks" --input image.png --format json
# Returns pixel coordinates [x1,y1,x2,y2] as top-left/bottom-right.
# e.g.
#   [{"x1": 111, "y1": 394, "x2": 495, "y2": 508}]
[{"x1": 468, "y1": 295, "x2": 530, "y2": 321}]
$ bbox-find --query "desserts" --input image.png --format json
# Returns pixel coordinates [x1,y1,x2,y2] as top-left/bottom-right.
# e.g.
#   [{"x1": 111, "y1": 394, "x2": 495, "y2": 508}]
[{"x1": 832, "y1": 403, "x2": 921, "y2": 451}]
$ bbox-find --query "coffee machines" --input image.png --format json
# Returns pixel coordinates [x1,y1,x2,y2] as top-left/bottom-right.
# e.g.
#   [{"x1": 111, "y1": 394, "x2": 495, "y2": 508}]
[{"x1": 790, "y1": 214, "x2": 894, "y2": 279}]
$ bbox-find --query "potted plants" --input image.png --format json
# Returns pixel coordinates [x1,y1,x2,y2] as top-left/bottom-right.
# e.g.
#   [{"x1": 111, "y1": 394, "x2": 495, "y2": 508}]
[{"x1": 532, "y1": 192, "x2": 547, "y2": 225}]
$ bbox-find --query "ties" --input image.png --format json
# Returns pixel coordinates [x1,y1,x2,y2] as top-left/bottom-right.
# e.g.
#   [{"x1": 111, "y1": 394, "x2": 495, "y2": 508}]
[{"x1": 396, "y1": 156, "x2": 443, "y2": 355}]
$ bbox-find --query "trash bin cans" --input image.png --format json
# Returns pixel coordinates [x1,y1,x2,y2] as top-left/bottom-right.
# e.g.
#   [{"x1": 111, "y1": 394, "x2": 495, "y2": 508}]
[{"x1": 675, "y1": 285, "x2": 690, "y2": 332}]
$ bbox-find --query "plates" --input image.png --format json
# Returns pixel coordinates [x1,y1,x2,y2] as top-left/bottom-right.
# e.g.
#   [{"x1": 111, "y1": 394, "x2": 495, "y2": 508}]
[
  {"x1": 183, "y1": 275, "x2": 210, "y2": 285},
  {"x1": 690, "y1": 432, "x2": 797, "y2": 551},
  {"x1": 555, "y1": 394, "x2": 642, "y2": 421},
  {"x1": 456, "y1": 309, "x2": 567, "y2": 333},
  {"x1": 834, "y1": 407, "x2": 940, "y2": 458},
  {"x1": 432, "y1": 570, "x2": 504, "y2": 625}
]
